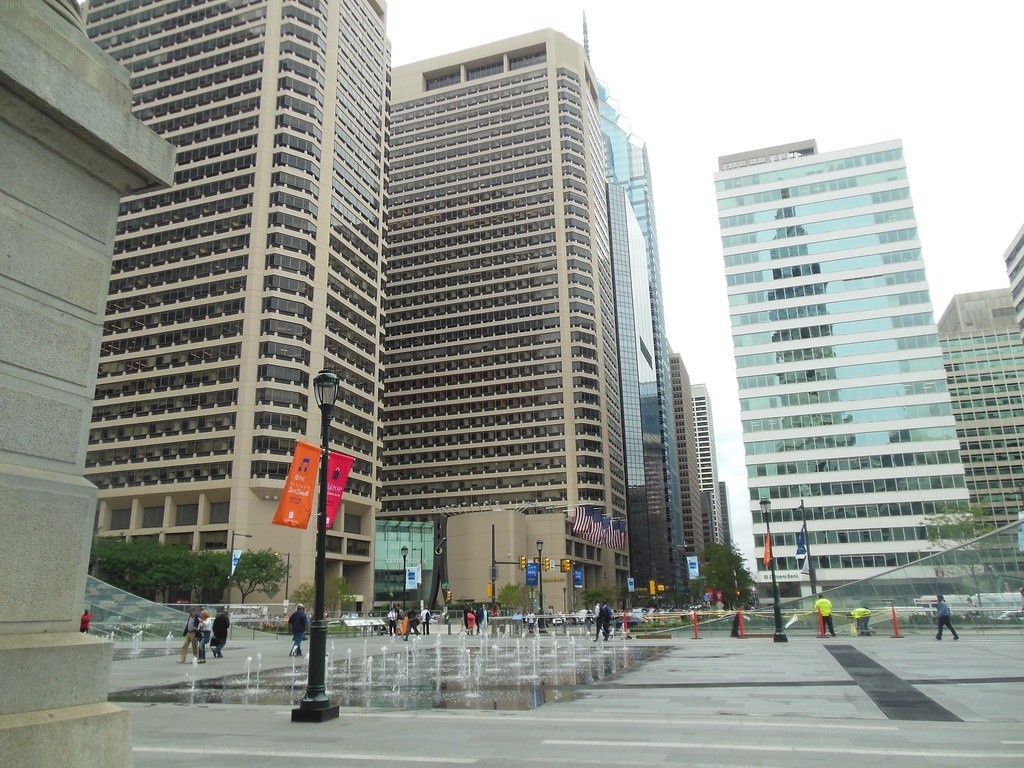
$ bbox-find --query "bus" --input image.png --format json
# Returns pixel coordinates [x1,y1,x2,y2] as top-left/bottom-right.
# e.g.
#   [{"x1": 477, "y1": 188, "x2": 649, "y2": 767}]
[{"x1": 914, "y1": 592, "x2": 1024, "y2": 608}]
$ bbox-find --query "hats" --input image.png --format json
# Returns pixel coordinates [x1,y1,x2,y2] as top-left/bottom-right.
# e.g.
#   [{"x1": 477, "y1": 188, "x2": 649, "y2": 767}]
[{"x1": 297, "y1": 604, "x2": 305, "y2": 609}]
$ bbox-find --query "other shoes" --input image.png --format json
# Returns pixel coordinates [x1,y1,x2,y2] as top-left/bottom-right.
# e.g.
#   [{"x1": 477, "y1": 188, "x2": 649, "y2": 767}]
[
  {"x1": 213, "y1": 649, "x2": 223, "y2": 658},
  {"x1": 403, "y1": 639, "x2": 408, "y2": 641},
  {"x1": 198, "y1": 659, "x2": 206, "y2": 662},
  {"x1": 289, "y1": 651, "x2": 297, "y2": 657},
  {"x1": 296, "y1": 649, "x2": 302, "y2": 655}
]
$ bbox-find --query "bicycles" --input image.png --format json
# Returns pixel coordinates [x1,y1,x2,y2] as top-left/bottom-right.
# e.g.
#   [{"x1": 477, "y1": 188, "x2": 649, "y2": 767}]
[{"x1": 235, "y1": 615, "x2": 291, "y2": 631}]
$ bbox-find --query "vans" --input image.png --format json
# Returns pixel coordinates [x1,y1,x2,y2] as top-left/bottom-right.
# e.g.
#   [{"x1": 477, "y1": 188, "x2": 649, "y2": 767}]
[{"x1": 996, "y1": 610, "x2": 1024, "y2": 619}]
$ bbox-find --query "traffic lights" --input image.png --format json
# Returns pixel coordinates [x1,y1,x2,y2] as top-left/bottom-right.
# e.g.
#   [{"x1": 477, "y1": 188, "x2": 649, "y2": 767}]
[
  {"x1": 447, "y1": 591, "x2": 452, "y2": 601},
  {"x1": 520, "y1": 555, "x2": 526, "y2": 571},
  {"x1": 545, "y1": 557, "x2": 550, "y2": 571},
  {"x1": 566, "y1": 559, "x2": 571, "y2": 571}
]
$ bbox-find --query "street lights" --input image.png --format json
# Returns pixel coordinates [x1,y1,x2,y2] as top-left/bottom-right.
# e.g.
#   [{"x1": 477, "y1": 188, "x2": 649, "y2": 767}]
[
  {"x1": 291, "y1": 367, "x2": 340, "y2": 723},
  {"x1": 536, "y1": 538, "x2": 549, "y2": 633},
  {"x1": 758, "y1": 496, "x2": 790, "y2": 642},
  {"x1": 275, "y1": 552, "x2": 290, "y2": 599},
  {"x1": 228, "y1": 529, "x2": 253, "y2": 604},
  {"x1": 401, "y1": 545, "x2": 410, "y2": 612},
  {"x1": 412, "y1": 549, "x2": 423, "y2": 600},
  {"x1": 563, "y1": 587, "x2": 567, "y2": 614}
]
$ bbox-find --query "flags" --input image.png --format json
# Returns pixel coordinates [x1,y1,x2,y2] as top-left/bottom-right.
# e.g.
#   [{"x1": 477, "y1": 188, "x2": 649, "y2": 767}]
[
  {"x1": 573, "y1": 506, "x2": 594, "y2": 533},
  {"x1": 613, "y1": 522, "x2": 625, "y2": 550},
  {"x1": 605, "y1": 520, "x2": 620, "y2": 548},
  {"x1": 592, "y1": 517, "x2": 610, "y2": 545},
  {"x1": 582, "y1": 510, "x2": 602, "y2": 541}
]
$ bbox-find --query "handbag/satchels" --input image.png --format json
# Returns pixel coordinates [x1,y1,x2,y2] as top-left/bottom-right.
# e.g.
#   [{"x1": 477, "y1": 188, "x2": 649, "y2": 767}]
[
  {"x1": 210, "y1": 636, "x2": 217, "y2": 646},
  {"x1": 426, "y1": 612, "x2": 430, "y2": 620}
]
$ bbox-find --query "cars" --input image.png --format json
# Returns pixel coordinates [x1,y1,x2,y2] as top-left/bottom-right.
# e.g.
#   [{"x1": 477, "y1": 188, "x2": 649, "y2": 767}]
[{"x1": 552, "y1": 604, "x2": 703, "y2": 626}]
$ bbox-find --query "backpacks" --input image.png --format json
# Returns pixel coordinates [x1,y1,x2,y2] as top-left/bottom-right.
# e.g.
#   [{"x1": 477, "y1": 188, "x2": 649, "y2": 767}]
[
  {"x1": 598, "y1": 605, "x2": 605, "y2": 618},
  {"x1": 605, "y1": 607, "x2": 612, "y2": 618}
]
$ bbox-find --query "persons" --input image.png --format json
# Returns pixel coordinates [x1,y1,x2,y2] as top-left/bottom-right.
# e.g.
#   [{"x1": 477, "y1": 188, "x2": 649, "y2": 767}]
[
  {"x1": 966, "y1": 597, "x2": 979, "y2": 618},
  {"x1": 585, "y1": 602, "x2": 613, "y2": 642},
  {"x1": 464, "y1": 603, "x2": 489, "y2": 636},
  {"x1": 288, "y1": 604, "x2": 307, "y2": 657},
  {"x1": 388, "y1": 605, "x2": 420, "y2": 640},
  {"x1": 545, "y1": 612, "x2": 549, "y2": 627},
  {"x1": 573, "y1": 610, "x2": 576, "y2": 626},
  {"x1": 847, "y1": 607, "x2": 871, "y2": 636},
  {"x1": 569, "y1": 609, "x2": 573, "y2": 625},
  {"x1": 176, "y1": 608, "x2": 230, "y2": 663},
  {"x1": 420, "y1": 607, "x2": 431, "y2": 635},
  {"x1": 491, "y1": 602, "x2": 499, "y2": 617},
  {"x1": 814, "y1": 595, "x2": 836, "y2": 637},
  {"x1": 518, "y1": 610, "x2": 535, "y2": 633},
  {"x1": 80, "y1": 609, "x2": 92, "y2": 633},
  {"x1": 933, "y1": 595, "x2": 959, "y2": 640}
]
